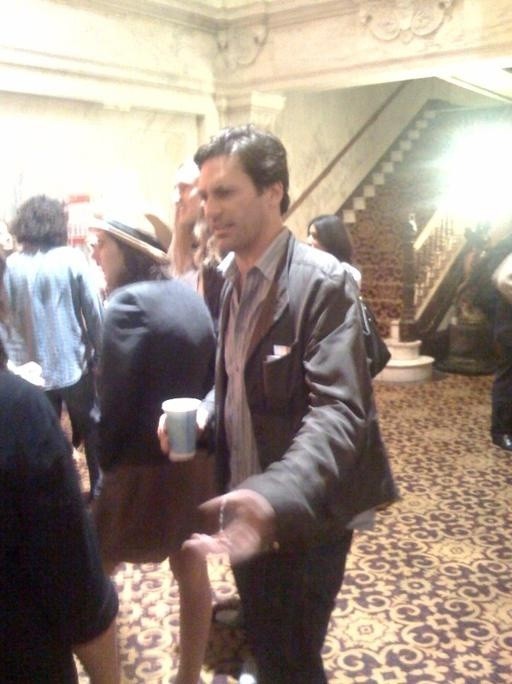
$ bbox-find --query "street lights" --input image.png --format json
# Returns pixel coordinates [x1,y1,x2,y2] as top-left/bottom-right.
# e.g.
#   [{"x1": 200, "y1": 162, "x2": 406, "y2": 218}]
[{"x1": 360, "y1": 297, "x2": 392, "y2": 382}]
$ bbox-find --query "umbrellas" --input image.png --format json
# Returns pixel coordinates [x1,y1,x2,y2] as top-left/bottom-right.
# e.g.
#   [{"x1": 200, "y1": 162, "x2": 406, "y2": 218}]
[{"x1": 492, "y1": 433, "x2": 512, "y2": 451}]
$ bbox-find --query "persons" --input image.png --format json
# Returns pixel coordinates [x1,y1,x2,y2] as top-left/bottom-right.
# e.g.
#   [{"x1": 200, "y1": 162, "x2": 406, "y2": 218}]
[
  {"x1": 302, "y1": 212, "x2": 393, "y2": 381},
  {"x1": 0, "y1": 351, "x2": 123, "y2": 684},
  {"x1": 1, "y1": 194, "x2": 104, "y2": 507},
  {"x1": 158, "y1": 128, "x2": 401, "y2": 684},
  {"x1": 70, "y1": 205, "x2": 212, "y2": 683},
  {"x1": 91, "y1": 157, "x2": 231, "y2": 339},
  {"x1": 2, "y1": 219, "x2": 13, "y2": 273},
  {"x1": 473, "y1": 236, "x2": 512, "y2": 451}
]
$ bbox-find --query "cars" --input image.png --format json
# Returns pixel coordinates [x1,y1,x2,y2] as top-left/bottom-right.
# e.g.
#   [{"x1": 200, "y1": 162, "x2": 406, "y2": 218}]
[{"x1": 160, "y1": 395, "x2": 203, "y2": 462}]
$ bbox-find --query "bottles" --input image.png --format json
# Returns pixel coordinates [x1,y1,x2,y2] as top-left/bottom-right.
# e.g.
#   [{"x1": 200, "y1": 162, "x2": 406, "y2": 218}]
[{"x1": 87, "y1": 209, "x2": 173, "y2": 263}]
